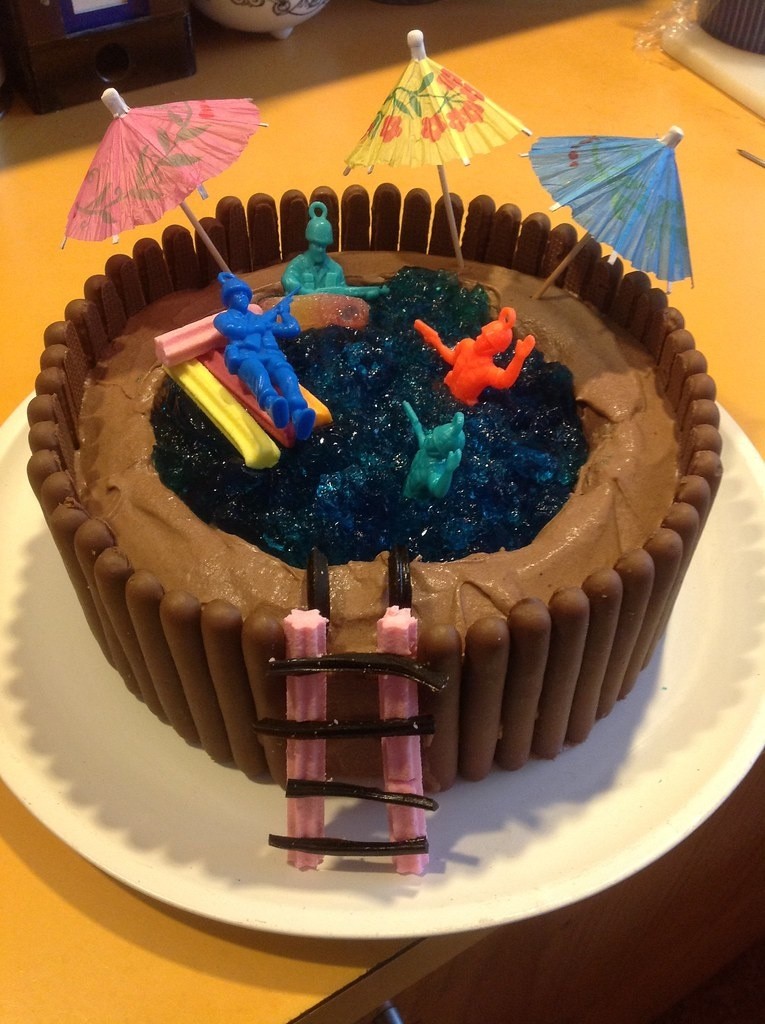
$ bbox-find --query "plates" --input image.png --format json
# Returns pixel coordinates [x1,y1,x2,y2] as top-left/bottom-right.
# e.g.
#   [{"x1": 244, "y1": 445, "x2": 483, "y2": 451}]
[{"x1": 0, "y1": 389, "x2": 765, "y2": 938}]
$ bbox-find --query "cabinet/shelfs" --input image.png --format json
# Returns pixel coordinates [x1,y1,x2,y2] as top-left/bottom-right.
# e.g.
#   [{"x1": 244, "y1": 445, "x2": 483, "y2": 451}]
[{"x1": 0, "y1": 0, "x2": 765, "y2": 1024}]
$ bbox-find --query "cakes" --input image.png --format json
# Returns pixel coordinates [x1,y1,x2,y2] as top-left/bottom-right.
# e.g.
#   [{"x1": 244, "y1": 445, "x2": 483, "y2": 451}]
[{"x1": 25, "y1": 178, "x2": 726, "y2": 870}]
